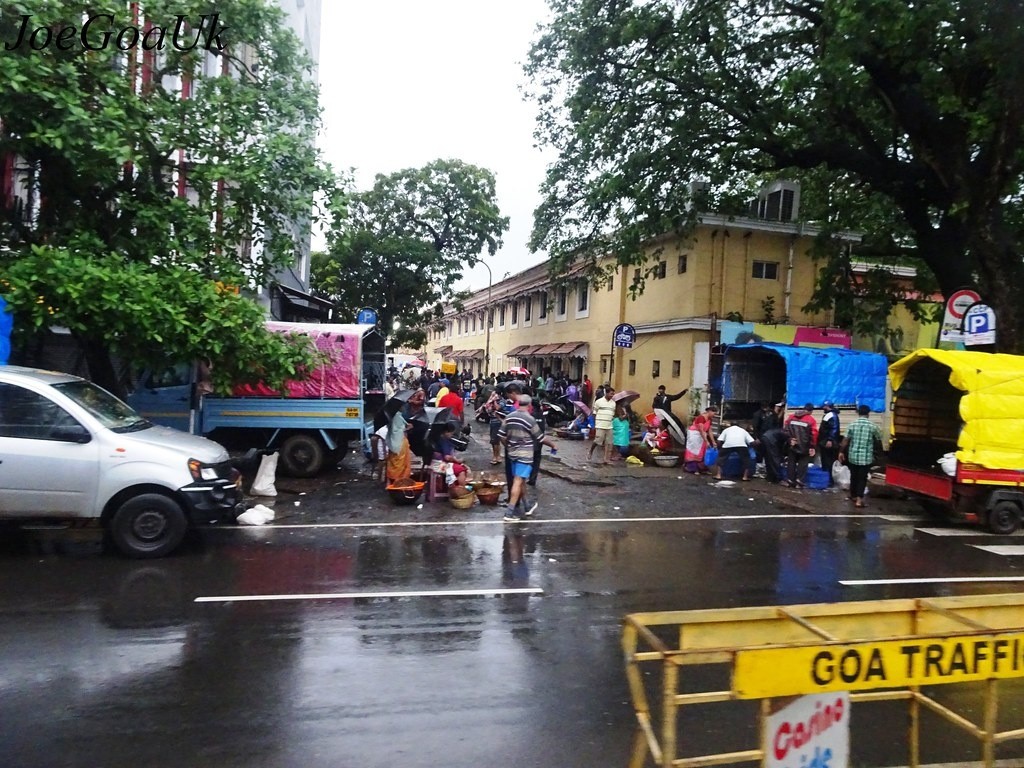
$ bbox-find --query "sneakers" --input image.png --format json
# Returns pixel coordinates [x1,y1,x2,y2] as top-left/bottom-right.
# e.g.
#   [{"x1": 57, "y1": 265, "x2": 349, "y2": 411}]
[
  {"x1": 525, "y1": 501, "x2": 538, "y2": 515},
  {"x1": 503, "y1": 512, "x2": 521, "y2": 521}
]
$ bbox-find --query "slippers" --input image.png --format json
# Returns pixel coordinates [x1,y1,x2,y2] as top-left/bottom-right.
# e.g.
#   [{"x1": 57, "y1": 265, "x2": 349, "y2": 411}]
[{"x1": 856, "y1": 502, "x2": 868, "y2": 507}]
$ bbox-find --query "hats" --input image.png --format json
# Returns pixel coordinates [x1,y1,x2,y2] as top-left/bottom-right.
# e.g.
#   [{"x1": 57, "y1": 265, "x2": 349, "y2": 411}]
[
  {"x1": 439, "y1": 378, "x2": 449, "y2": 386},
  {"x1": 820, "y1": 400, "x2": 832, "y2": 408}
]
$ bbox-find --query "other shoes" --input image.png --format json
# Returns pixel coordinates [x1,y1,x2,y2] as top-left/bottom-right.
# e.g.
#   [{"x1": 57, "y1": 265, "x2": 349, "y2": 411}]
[
  {"x1": 585, "y1": 452, "x2": 592, "y2": 462},
  {"x1": 490, "y1": 458, "x2": 502, "y2": 464},
  {"x1": 702, "y1": 470, "x2": 713, "y2": 475},
  {"x1": 693, "y1": 471, "x2": 700, "y2": 475},
  {"x1": 742, "y1": 478, "x2": 750, "y2": 481},
  {"x1": 713, "y1": 476, "x2": 721, "y2": 480},
  {"x1": 603, "y1": 458, "x2": 613, "y2": 464}
]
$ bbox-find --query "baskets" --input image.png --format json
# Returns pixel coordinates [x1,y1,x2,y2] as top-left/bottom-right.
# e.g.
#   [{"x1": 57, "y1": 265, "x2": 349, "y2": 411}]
[
  {"x1": 389, "y1": 487, "x2": 422, "y2": 506},
  {"x1": 449, "y1": 483, "x2": 475, "y2": 508}
]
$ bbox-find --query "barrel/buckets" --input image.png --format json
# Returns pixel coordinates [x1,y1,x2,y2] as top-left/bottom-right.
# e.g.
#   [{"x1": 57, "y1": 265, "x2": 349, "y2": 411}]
[
  {"x1": 720, "y1": 447, "x2": 757, "y2": 478},
  {"x1": 581, "y1": 429, "x2": 590, "y2": 439}
]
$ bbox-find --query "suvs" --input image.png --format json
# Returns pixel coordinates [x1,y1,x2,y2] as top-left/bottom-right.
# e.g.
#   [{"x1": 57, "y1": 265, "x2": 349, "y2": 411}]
[{"x1": 0, "y1": 365, "x2": 283, "y2": 563}]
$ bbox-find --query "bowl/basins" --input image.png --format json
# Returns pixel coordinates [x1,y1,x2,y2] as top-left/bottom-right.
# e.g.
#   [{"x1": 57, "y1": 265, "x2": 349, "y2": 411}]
[
  {"x1": 560, "y1": 428, "x2": 567, "y2": 431},
  {"x1": 567, "y1": 431, "x2": 582, "y2": 438},
  {"x1": 557, "y1": 431, "x2": 568, "y2": 437},
  {"x1": 654, "y1": 456, "x2": 680, "y2": 467}
]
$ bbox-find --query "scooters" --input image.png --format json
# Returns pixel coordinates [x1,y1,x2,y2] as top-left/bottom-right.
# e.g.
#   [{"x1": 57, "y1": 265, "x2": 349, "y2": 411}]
[
  {"x1": 472, "y1": 392, "x2": 490, "y2": 423},
  {"x1": 540, "y1": 394, "x2": 573, "y2": 427}
]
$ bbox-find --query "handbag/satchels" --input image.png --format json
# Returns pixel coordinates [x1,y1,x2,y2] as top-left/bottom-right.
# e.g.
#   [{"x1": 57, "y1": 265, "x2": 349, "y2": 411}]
[
  {"x1": 704, "y1": 446, "x2": 719, "y2": 465},
  {"x1": 831, "y1": 460, "x2": 851, "y2": 489}
]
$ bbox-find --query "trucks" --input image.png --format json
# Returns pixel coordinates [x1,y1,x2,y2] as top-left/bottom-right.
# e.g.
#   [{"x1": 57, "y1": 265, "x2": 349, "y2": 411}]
[
  {"x1": 720, "y1": 342, "x2": 907, "y2": 469},
  {"x1": 885, "y1": 348, "x2": 1024, "y2": 534},
  {"x1": 124, "y1": 323, "x2": 388, "y2": 478}
]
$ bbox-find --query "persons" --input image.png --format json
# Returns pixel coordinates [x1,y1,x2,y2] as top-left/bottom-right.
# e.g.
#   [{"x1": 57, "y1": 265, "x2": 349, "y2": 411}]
[{"x1": 367, "y1": 364, "x2": 883, "y2": 522}]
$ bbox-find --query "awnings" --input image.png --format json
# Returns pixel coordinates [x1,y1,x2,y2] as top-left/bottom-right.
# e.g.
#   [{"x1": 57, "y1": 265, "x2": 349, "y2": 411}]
[
  {"x1": 504, "y1": 342, "x2": 588, "y2": 359},
  {"x1": 400, "y1": 351, "x2": 427, "y2": 359},
  {"x1": 445, "y1": 349, "x2": 484, "y2": 361}
]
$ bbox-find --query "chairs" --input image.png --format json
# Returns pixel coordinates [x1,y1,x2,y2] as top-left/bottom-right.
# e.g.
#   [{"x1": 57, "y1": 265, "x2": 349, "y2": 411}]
[{"x1": 369, "y1": 433, "x2": 388, "y2": 480}]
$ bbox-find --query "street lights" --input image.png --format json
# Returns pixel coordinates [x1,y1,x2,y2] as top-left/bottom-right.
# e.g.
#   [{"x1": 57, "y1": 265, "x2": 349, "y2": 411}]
[{"x1": 458, "y1": 252, "x2": 493, "y2": 381}]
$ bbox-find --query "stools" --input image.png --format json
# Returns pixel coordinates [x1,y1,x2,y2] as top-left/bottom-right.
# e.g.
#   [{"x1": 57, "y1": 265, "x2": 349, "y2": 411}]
[{"x1": 420, "y1": 468, "x2": 449, "y2": 502}]
